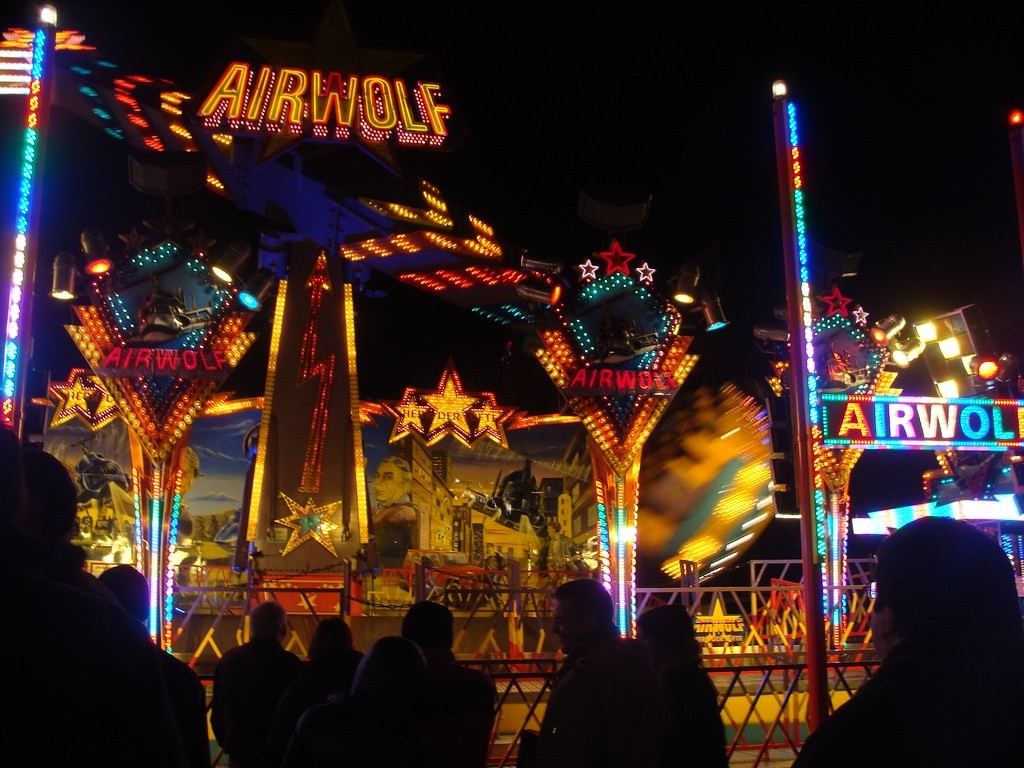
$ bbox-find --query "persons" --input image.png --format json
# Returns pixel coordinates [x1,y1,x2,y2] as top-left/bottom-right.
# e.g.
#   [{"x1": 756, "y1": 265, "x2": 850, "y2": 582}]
[
  {"x1": 100, "y1": 565, "x2": 212, "y2": 768},
  {"x1": 0, "y1": 427, "x2": 189, "y2": 768},
  {"x1": 17, "y1": 447, "x2": 121, "y2": 609},
  {"x1": 539, "y1": 579, "x2": 678, "y2": 768},
  {"x1": 268, "y1": 618, "x2": 364, "y2": 758},
  {"x1": 636, "y1": 606, "x2": 730, "y2": 768},
  {"x1": 212, "y1": 602, "x2": 302, "y2": 768},
  {"x1": 790, "y1": 516, "x2": 1024, "y2": 768},
  {"x1": 402, "y1": 602, "x2": 494, "y2": 768},
  {"x1": 282, "y1": 637, "x2": 438, "y2": 768}
]
